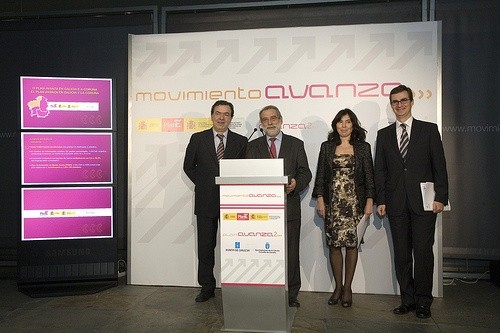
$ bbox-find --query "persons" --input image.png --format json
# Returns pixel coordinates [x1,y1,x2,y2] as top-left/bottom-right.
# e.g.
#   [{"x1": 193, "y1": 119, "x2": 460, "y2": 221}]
[
  {"x1": 312, "y1": 108, "x2": 374, "y2": 307},
  {"x1": 184, "y1": 100, "x2": 248, "y2": 305},
  {"x1": 374, "y1": 85, "x2": 449, "y2": 319},
  {"x1": 247, "y1": 106, "x2": 312, "y2": 307}
]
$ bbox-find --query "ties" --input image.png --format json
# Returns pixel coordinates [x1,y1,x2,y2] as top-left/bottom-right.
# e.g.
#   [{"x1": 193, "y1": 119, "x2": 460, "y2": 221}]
[
  {"x1": 269, "y1": 138, "x2": 277, "y2": 159},
  {"x1": 399, "y1": 124, "x2": 409, "y2": 159},
  {"x1": 216, "y1": 134, "x2": 225, "y2": 160}
]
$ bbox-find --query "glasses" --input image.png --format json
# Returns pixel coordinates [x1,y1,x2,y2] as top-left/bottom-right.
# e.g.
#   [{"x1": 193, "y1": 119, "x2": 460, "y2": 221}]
[
  {"x1": 215, "y1": 111, "x2": 231, "y2": 118},
  {"x1": 263, "y1": 116, "x2": 280, "y2": 123},
  {"x1": 391, "y1": 98, "x2": 410, "y2": 106}
]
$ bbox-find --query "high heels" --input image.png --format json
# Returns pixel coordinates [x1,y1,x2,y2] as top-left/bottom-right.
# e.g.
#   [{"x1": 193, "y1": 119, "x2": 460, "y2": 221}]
[
  {"x1": 341, "y1": 289, "x2": 352, "y2": 307},
  {"x1": 328, "y1": 286, "x2": 344, "y2": 305}
]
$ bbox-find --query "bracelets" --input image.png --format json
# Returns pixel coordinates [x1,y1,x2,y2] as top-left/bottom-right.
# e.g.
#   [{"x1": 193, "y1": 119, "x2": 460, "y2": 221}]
[{"x1": 316, "y1": 196, "x2": 323, "y2": 198}]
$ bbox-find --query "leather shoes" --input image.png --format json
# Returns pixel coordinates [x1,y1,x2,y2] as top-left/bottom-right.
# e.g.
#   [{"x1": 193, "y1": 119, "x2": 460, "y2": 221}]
[
  {"x1": 415, "y1": 304, "x2": 431, "y2": 318},
  {"x1": 392, "y1": 304, "x2": 416, "y2": 314}
]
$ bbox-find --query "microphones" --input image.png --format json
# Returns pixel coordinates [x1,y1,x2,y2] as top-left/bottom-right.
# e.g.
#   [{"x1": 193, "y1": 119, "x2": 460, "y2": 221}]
[
  {"x1": 237, "y1": 128, "x2": 257, "y2": 158},
  {"x1": 260, "y1": 128, "x2": 274, "y2": 158}
]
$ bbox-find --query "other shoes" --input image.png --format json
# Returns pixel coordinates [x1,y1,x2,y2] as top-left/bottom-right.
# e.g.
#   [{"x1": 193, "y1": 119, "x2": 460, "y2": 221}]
[
  {"x1": 289, "y1": 300, "x2": 300, "y2": 308},
  {"x1": 195, "y1": 291, "x2": 215, "y2": 302}
]
